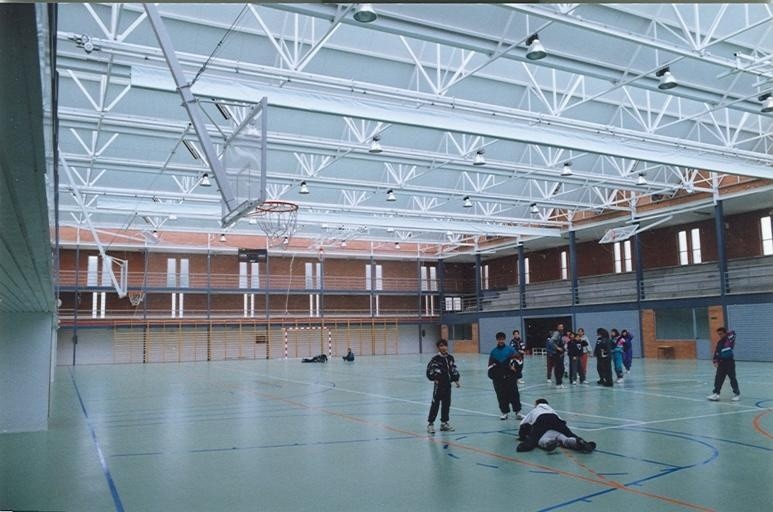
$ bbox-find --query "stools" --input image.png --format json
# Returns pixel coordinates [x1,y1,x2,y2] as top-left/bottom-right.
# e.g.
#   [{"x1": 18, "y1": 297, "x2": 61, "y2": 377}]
[{"x1": 656, "y1": 345, "x2": 673, "y2": 361}]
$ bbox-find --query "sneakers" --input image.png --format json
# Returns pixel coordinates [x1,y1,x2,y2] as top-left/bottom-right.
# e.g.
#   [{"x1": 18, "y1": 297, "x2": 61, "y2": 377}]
[
  {"x1": 427, "y1": 423, "x2": 435, "y2": 433},
  {"x1": 732, "y1": 393, "x2": 743, "y2": 401},
  {"x1": 706, "y1": 393, "x2": 719, "y2": 401},
  {"x1": 516, "y1": 413, "x2": 525, "y2": 420},
  {"x1": 440, "y1": 423, "x2": 454, "y2": 431},
  {"x1": 548, "y1": 369, "x2": 631, "y2": 390},
  {"x1": 500, "y1": 414, "x2": 508, "y2": 419}
]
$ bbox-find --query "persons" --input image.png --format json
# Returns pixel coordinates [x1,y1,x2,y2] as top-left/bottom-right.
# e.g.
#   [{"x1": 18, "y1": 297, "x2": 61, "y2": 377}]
[
  {"x1": 424, "y1": 338, "x2": 461, "y2": 434},
  {"x1": 486, "y1": 331, "x2": 526, "y2": 420},
  {"x1": 508, "y1": 329, "x2": 525, "y2": 384},
  {"x1": 704, "y1": 327, "x2": 743, "y2": 403},
  {"x1": 515, "y1": 399, "x2": 598, "y2": 453},
  {"x1": 300, "y1": 354, "x2": 328, "y2": 364},
  {"x1": 341, "y1": 347, "x2": 354, "y2": 362},
  {"x1": 543, "y1": 323, "x2": 634, "y2": 389}
]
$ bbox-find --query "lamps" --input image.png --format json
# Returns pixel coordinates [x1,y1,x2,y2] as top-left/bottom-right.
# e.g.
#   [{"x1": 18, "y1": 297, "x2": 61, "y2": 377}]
[
  {"x1": 442, "y1": 173, "x2": 654, "y2": 260},
  {"x1": 215, "y1": 29, "x2": 548, "y2": 250},
  {"x1": 389, "y1": 88, "x2": 773, "y2": 267},
  {"x1": 139, "y1": 3, "x2": 377, "y2": 246},
  {"x1": 312, "y1": 61, "x2": 682, "y2": 248}
]
